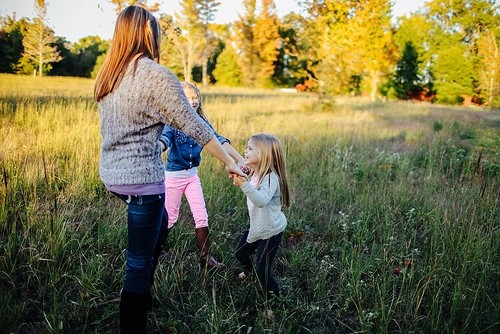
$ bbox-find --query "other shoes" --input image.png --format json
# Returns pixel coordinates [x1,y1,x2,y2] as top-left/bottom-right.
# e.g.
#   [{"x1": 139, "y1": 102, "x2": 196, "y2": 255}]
[
  {"x1": 263, "y1": 306, "x2": 279, "y2": 319},
  {"x1": 238, "y1": 271, "x2": 252, "y2": 279}
]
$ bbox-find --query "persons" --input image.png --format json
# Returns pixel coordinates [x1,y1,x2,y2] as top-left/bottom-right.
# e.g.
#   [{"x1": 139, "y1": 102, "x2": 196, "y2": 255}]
[
  {"x1": 93, "y1": 5, "x2": 250, "y2": 334},
  {"x1": 158, "y1": 80, "x2": 252, "y2": 270},
  {"x1": 233, "y1": 133, "x2": 290, "y2": 308}
]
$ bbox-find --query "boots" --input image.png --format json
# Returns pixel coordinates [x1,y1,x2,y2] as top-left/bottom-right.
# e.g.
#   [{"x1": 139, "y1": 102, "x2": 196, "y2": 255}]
[
  {"x1": 130, "y1": 244, "x2": 163, "y2": 308},
  {"x1": 195, "y1": 226, "x2": 227, "y2": 270},
  {"x1": 119, "y1": 297, "x2": 162, "y2": 334}
]
